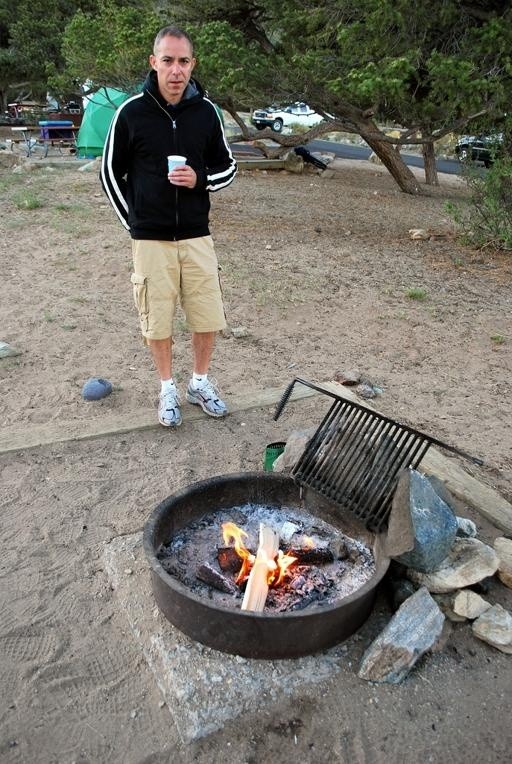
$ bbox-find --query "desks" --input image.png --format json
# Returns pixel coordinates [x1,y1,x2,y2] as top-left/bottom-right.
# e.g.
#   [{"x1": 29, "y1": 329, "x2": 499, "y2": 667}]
[{"x1": 10, "y1": 126, "x2": 82, "y2": 159}]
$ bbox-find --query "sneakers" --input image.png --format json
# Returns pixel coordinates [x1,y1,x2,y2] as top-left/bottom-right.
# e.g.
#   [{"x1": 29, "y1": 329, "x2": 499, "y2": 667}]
[
  {"x1": 185, "y1": 379, "x2": 228, "y2": 418},
  {"x1": 157, "y1": 389, "x2": 182, "y2": 427}
]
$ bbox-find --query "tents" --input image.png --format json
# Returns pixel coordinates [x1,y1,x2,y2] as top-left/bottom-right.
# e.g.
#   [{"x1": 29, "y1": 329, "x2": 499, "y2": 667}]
[{"x1": 76, "y1": 81, "x2": 225, "y2": 157}]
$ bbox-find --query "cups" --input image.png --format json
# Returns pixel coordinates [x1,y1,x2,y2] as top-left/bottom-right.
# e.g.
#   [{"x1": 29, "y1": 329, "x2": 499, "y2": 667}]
[{"x1": 167, "y1": 153, "x2": 185, "y2": 180}]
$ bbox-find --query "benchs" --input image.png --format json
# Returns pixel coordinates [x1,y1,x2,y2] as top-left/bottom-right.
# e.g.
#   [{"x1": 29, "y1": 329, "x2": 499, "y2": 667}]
[
  {"x1": 34, "y1": 137, "x2": 79, "y2": 159},
  {"x1": 6, "y1": 138, "x2": 44, "y2": 159}
]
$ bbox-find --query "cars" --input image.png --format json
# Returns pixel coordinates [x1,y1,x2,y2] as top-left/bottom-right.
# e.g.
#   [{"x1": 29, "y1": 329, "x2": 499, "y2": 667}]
[{"x1": 456, "y1": 130, "x2": 504, "y2": 169}]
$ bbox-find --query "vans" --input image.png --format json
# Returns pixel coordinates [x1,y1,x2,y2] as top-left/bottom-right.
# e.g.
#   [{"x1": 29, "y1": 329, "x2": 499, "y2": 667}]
[{"x1": 249, "y1": 97, "x2": 335, "y2": 132}]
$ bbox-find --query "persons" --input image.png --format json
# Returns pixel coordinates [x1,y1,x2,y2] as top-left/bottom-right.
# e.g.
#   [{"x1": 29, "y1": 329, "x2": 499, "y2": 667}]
[{"x1": 100, "y1": 27, "x2": 238, "y2": 428}]
